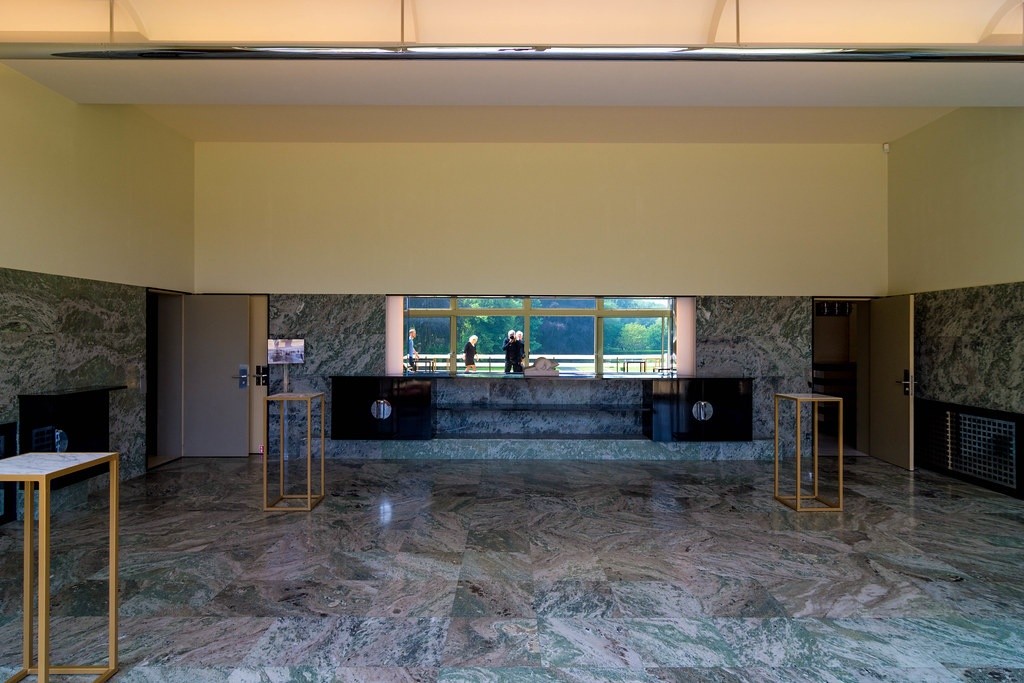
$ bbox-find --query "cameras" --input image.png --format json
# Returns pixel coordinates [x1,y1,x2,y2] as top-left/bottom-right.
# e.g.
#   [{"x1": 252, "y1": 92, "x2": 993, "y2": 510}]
[{"x1": 510, "y1": 336, "x2": 514, "y2": 340}]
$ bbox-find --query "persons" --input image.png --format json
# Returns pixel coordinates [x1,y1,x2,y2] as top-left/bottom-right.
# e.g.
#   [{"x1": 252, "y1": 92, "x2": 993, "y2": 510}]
[
  {"x1": 463, "y1": 335, "x2": 479, "y2": 372},
  {"x1": 409, "y1": 330, "x2": 419, "y2": 372},
  {"x1": 502, "y1": 329, "x2": 524, "y2": 373}
]
